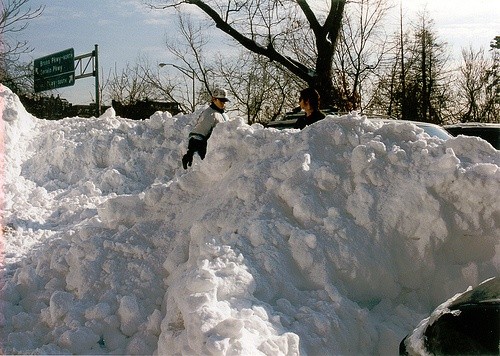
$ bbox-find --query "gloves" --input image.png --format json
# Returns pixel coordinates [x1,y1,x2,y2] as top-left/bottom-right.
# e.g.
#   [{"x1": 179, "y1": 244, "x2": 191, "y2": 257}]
[{"x1": 182, "y1": 152, "x2": 193, "y2": 170}]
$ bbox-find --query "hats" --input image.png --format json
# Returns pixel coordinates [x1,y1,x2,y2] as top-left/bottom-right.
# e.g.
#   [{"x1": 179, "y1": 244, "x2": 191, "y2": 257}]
[{"x1": 212, "y1": 88, "x2": 229, "y2": 102}]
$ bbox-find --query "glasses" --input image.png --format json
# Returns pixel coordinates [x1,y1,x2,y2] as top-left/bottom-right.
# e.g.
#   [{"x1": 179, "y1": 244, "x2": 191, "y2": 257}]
[
  {"x1": 217, "y1": 98, "x2": 226, "y2": 103},
  {"x1": 299, "y1": 97, "x2": 303, "y2": 102}
]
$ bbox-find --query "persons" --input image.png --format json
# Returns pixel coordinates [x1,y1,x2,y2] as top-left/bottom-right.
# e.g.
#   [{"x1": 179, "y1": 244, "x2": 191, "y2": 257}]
[
  {"x1": 182, "y1": 87, "x2": 231, "y2": 170},
  {"x1": 293, "y1": 88, "x2": 327, "y2": 130}
]
